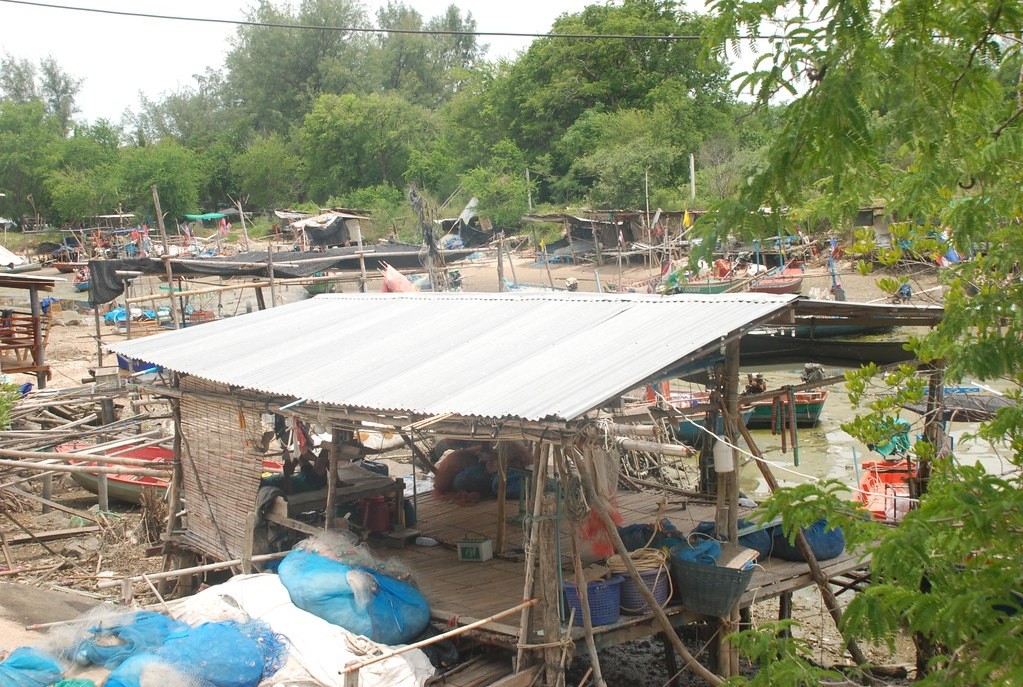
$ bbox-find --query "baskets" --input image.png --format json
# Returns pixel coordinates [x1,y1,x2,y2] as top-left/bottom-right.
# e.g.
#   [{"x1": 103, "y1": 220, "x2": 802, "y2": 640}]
[
  {"x1": 674, "y1": 540, "x2": 760, "y2": 617},
  {"x1": 608, "y1": 564, "x2": 671, "y2": 614},
  {"x1": 558, "y1": 573, "x2": 626, "y2": 625}
]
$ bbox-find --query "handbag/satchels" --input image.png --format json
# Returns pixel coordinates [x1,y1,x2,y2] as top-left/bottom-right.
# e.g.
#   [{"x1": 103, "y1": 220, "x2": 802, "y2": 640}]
[{"x1": 0, "y1": 647, "x2": 63, "y2": 687}]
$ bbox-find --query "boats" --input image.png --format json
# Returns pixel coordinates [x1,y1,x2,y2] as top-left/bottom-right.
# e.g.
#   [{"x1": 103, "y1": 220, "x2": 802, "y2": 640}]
[
  {"x1": 687, "y1": 389, "x2": 831, "y2": 430},
  {"x1": 0, "y1": 225, "x2": 807, "y2": 326},
  {"x1": 622, "y1": 396, "x2": 757, "y2": 445},
  {"x1": 853, "y1": 455, "x2": 921, "y2": 532},
  {"x1": 56, "y1": 433, "x2": 288, "y2": 510}
]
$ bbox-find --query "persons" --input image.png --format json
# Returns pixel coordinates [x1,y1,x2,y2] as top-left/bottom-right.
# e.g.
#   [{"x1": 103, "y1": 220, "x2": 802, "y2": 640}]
[{"x1": 298, "y1": 448, "x2": 331, "y2": 490}]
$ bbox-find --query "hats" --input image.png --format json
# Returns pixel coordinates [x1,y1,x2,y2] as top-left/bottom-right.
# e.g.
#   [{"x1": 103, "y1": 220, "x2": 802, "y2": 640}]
[{"x1": 753, "y1": 374, "x2": 766, "y2": 380}]
